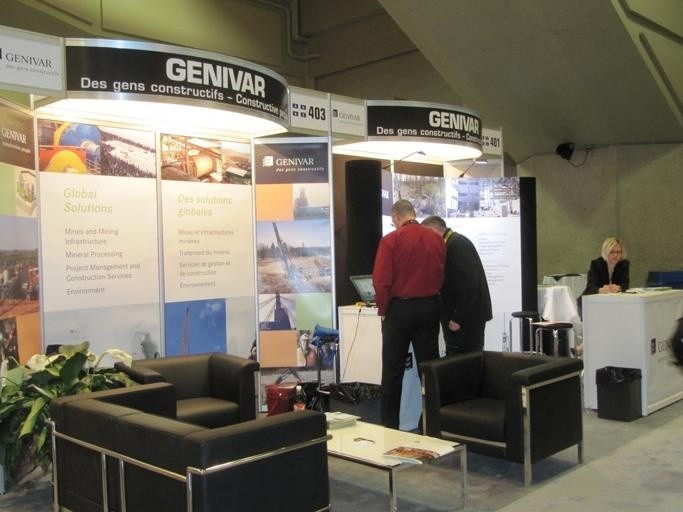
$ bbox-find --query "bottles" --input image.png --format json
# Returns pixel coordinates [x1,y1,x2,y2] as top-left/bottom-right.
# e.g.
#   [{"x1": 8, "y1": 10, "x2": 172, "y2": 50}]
[{"x1": 292, "y1": 385, "x2": 305, "y2": 412}]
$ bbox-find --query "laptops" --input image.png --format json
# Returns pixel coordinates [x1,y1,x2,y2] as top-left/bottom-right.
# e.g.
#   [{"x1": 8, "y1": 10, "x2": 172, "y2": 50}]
[{"x1": 349, "y1": 274, "x2": 377, "y2": 307}]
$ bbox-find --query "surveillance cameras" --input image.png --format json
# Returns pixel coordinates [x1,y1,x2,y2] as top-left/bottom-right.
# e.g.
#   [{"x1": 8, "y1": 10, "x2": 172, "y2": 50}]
[{"x1": 556, "y1": 142, "x2": 575, "y2": 160}]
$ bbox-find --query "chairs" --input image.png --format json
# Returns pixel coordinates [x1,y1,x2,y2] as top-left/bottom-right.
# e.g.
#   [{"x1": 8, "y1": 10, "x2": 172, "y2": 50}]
[
  {"x1": 418, "y1": 350, "x2": 586, "y2": 487},
  {"x1": 115, "y1": 352, "x2": 260, "y2": 429},
  {"x1": 45, "y1": 380, "x2": 333, "y2": 512}
]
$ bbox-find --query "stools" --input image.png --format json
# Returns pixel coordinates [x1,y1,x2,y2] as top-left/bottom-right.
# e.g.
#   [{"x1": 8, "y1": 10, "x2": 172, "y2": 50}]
[
  {"x1": 531, "y1": 322, "x2": 573, "y2": 356},
  {"x1": 316, "y1": 337, "x2": 340, "y2": 395},
  {"x1": 509, "y1": 311, "x2": 539, "y2": 353}
]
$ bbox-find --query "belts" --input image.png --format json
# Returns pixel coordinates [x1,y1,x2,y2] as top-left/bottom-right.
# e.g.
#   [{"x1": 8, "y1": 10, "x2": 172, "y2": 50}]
[{"x1": 395, "y1": 295, "x2": 435, "y2": 302}]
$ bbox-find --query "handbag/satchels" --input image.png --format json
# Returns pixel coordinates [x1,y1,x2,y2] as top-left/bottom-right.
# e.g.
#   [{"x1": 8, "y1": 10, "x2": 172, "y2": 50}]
[
  {"x1": 289, "y1": 383, "x2": 330, "y2": 413},
  {"x1": 266, "y1": 381, "x2": 298, "y2": 416}
]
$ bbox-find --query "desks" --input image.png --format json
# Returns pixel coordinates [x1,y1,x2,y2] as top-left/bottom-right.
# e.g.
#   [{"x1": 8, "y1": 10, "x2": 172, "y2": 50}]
[
  {"x1": 338, "y1": 304, "x2": 383, "y2": 386},
  {"x1": 581, "y1": 289, "x2": 683, "y2": 416},
  {"x1": 537, "y1": 273, "x2": 588, "y2": 356}
]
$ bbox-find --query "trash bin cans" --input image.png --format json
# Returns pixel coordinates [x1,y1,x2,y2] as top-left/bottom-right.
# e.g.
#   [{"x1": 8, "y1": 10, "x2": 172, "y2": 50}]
[
  {"x1": 596, "y1": 365, "x2": 642, "y2": 423},
  {"x1": 330, "y1": 381, "x2": 381, "y2": 424}
]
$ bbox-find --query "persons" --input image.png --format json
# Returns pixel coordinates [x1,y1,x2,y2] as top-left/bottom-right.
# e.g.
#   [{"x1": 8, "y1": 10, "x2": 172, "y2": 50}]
[
  {"x1": 375, "y1": 202, "x2": 447, "y2": 431},
  {"x1": 417, "y1": 213, "x2": 492, "y2": 354},
  {"x1": 569, "y1": 236, "x2": 629, "y2": 357}
]
